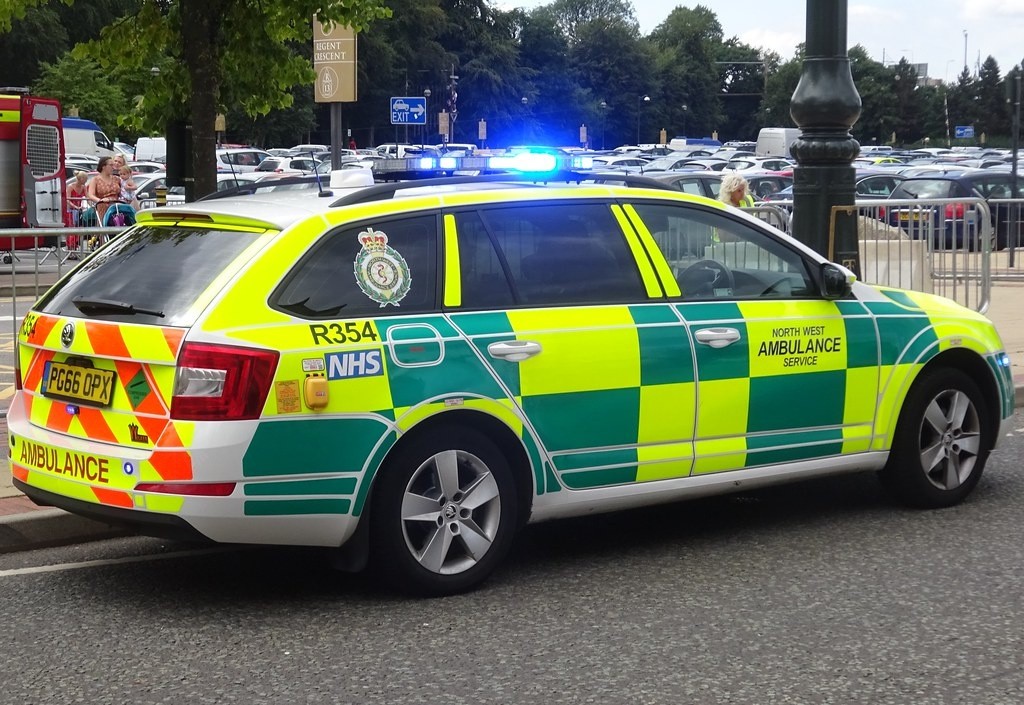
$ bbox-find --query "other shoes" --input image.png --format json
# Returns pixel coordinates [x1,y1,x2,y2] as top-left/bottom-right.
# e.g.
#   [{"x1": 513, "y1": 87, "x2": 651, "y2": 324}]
[{"x1": 66, "y1": 252, "x2": 77, "y2": 260}]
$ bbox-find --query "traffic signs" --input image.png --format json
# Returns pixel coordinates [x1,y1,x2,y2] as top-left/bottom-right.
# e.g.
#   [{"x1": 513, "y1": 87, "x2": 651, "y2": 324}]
[
  {"x1": 390, "y1": 96, "x2": 428, "y2": 124},
  {"x1": 955, "y1": 126, "x2": 974, "y2": 138}
]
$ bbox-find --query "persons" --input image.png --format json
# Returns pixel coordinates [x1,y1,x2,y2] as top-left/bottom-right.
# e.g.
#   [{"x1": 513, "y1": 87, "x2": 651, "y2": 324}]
[
  {"x1": 713, "y1": 173, "x2": 756, "y2": 243},
  {"x1": 65, "y1": 153, "x2": 138, "y2": 244}
]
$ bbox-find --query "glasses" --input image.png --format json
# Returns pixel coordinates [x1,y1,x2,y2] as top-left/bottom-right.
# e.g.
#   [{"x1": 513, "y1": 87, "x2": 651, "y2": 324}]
[
  {"x1": 105, "y1": 164, "x2": 113, "y2": 167},
  {"x1": 737, "y1": 188, "x2": 744, "y2": 191}
]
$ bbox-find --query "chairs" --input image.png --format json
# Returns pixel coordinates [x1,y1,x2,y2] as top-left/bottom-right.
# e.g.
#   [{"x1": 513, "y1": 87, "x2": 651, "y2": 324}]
[
  {"x1": 761, "y1": 182, "x2": 773, "y2": 194},
  {"x1": 991, "y1": 185, "x2": 1006, "y2": 198},
  {"x1": 870, "y1": 180, "x2": 886, "y2": 194}
]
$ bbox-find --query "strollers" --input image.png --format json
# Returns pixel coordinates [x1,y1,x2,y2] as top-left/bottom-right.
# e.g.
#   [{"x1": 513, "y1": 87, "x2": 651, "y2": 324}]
[
  {"x1": 94, "y1": 200, "x2": 138, "y2": 244},
  {"x1": 78, "y1": 205, "x2": 98, "y2": 259}
]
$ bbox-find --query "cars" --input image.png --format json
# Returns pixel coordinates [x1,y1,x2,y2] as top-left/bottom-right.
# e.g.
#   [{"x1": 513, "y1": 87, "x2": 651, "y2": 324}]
[{"x1": 64, "y1": 138, "x2": 1023, "y2": 256}]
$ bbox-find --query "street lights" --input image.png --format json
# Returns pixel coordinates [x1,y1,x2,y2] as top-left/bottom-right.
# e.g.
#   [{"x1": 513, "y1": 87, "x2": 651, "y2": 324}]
[
  {"x1": 601, "y1": 97, "x2": 607, "y2": 150},
  {"x1": 522, "y1": 94, "x2": 529, "y2": 146},
  {"x1": 638, "y1": 95, "x2": 651, "y2": 145},
  {"x1": 681, "y1": 105, "x2": 687, "y2": 137}
]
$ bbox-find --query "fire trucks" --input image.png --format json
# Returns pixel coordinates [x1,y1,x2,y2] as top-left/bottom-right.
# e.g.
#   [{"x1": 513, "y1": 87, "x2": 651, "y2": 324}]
[{"x1": 1, "y1": 88, "x2": 68, "y2": 251}]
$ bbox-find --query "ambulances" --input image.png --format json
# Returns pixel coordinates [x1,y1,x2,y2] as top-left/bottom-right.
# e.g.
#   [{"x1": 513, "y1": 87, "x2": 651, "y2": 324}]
[{"x1": 5, "y1": 170, "x2": 1011, "y2": 601}]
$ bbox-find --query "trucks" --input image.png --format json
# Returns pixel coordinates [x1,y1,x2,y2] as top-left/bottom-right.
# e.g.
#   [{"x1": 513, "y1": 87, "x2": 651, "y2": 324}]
[{"x1": 59, "y1": 116, "x2": 127, "y2": 162}]
